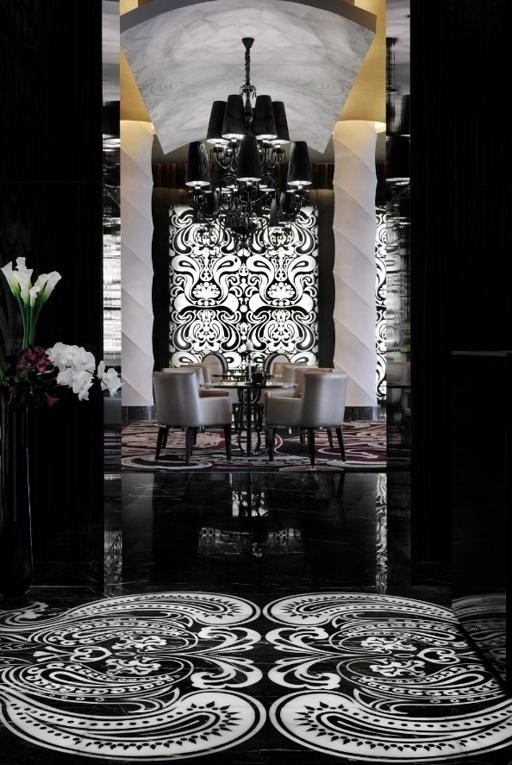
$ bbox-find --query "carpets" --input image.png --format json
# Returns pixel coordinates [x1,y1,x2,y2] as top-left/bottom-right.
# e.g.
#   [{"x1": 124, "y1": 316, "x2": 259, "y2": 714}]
[
  {"x1": 121, "y1": 418, "x2": 386, "y2": 472},
  {"x1": 385, "y1": 424, "x2": 411, "y2": 469},
  {"x1": 104, "y1": 431, "x2": 119, "y2": 473}
]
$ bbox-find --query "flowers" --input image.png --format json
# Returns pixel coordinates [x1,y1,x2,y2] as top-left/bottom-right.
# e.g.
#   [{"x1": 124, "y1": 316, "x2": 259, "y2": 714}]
[{"x1": 2, "y1": 256, "x2": 126, "y2": 413}]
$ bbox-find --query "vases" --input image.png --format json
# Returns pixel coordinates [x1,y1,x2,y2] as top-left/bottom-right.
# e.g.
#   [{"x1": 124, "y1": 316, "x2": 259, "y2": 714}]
[{"x1": 2, "y1": 384, "x2": 32, "y2": 609}]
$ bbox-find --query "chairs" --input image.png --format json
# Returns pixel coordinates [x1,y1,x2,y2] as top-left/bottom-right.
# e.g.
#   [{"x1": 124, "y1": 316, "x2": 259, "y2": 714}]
[
  {"x1": 104, "y1": 391, "x2": 121, "y2": 424},
  {"x1": 152, "y1": 350, "x2": 348, "y2": 468},
  {"x1": 386, "y1": 361, "x2": 411, "y2": 420}
]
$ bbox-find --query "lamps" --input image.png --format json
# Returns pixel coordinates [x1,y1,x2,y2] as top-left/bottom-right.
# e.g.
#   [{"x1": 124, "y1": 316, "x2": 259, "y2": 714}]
[{"x1": 185, "y1": 37, "x2": 314, "y2": 261}]
